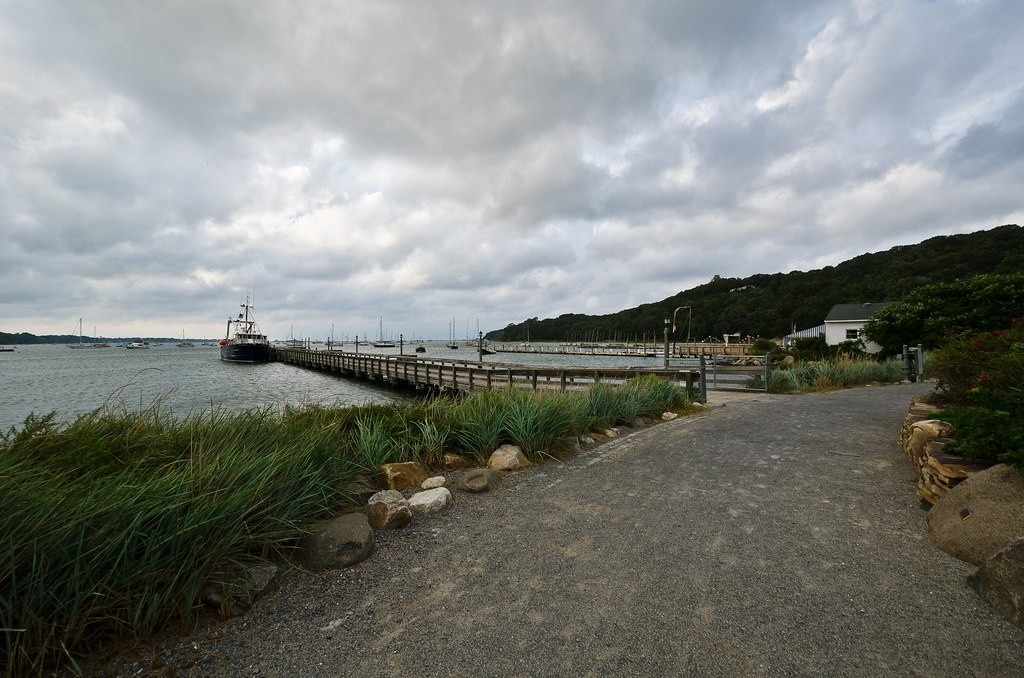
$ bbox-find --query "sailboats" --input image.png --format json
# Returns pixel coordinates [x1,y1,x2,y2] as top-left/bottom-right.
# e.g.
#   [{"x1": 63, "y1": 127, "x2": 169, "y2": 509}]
[
  {"x1": 65, "y1": 318, "x2": 92, "y2": 349},
  {"x1": 446, "y1": 316, "x2": 458, "y2": 349},
  {"x1": 374, "y1": 316, "x2": 396, "y2": 347}
]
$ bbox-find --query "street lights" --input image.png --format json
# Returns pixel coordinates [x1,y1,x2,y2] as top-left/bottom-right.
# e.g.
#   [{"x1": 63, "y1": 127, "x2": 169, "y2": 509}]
[
  {"x1": 479, "y1": 331, "x2": 483, "y2": 368},
  {"x1": 328, "y1": 337, "x2": 329, "y2": 350},
  {"x1": 356, "y1": 336, "x2": 358, "y2": 352},
  {"x1": 663, "y1": 317, "x2": 671, "y2": 366},
  {"x1": 400, "y1": 334, "x2": 403, "y2": 356}
]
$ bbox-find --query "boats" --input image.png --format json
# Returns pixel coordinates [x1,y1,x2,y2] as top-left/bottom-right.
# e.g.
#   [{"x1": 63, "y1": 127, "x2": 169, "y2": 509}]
[
  {"x1": 477, "y1": 348, "x2": 497, "y2": 354},
  {"x1": 220, "y1": 296, "x2": 270, "y2": 363},
  {"x1": 416, "y1": 346, "x2": 426, "y2": 353},
  {"x1": 126, "y1": 343, "x2": 149, "y2": 349}
]
